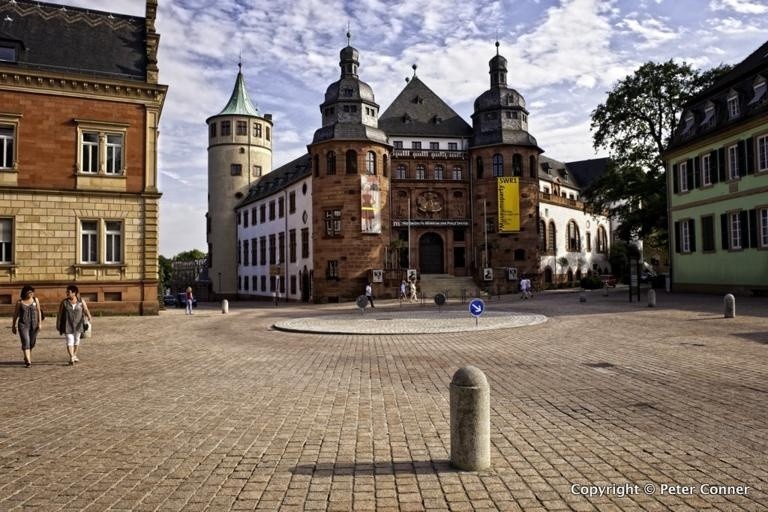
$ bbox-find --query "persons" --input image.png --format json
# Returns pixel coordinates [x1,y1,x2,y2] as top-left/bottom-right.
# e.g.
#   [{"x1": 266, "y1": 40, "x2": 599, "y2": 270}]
[
  {"x1": 408, "y1": 274, "x2": 415, "y2": 286},
  {"x1": 400, "y1": 280, "x2": 408, "y2": 303},
  {"x1": 12, "y1": 285, "x2": 42, "y2": 366},
  {"x1": 185, "y1": 287, "x2": 195, "y2": 314},
  {"x1": 365, "y1": 282, "x2": 375, "y2": 308},
  {"x1": 409, "y1": 277, "x2": 419, "y2": 303},
  {"x1": 524, "y1": 276, "x2": 534, "y2": 299},
  {"x1": 642, "y1": 259, "x2": 651, "y2": 277},
  {"x1": 55, "y1": 285, "x2": 91, "y2": 364},
  {"x1": 520, "y1": 276, "x2": 529, "y2": 299}
]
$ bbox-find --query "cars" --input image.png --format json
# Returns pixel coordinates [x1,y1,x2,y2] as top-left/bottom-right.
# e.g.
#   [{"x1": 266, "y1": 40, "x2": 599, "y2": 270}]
[
  {"x1": 175, "y1": 292, "x2": 199, "y2": 308},
  {"x1": 163, "y1": 294, "x2": 177, "y2": 307}
]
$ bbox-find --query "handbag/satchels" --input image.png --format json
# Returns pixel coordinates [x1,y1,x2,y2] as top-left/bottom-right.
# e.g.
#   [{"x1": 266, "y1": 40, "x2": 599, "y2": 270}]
[
  {"x1": 81, "y1": 317, "x2": 91, "y2": 338},
  {"x1": 38, "y1": 308, "x2": 44, "y2": 322}
]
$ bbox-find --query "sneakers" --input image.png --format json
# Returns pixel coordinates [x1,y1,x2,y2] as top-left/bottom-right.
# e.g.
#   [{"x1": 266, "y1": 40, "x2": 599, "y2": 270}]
[
  {"x1": 69, "y1": 354, "x2": 79, "y2": 363},
  {"x1": 26, "y1": 364, "x2": 32, "y2": 369}
]
